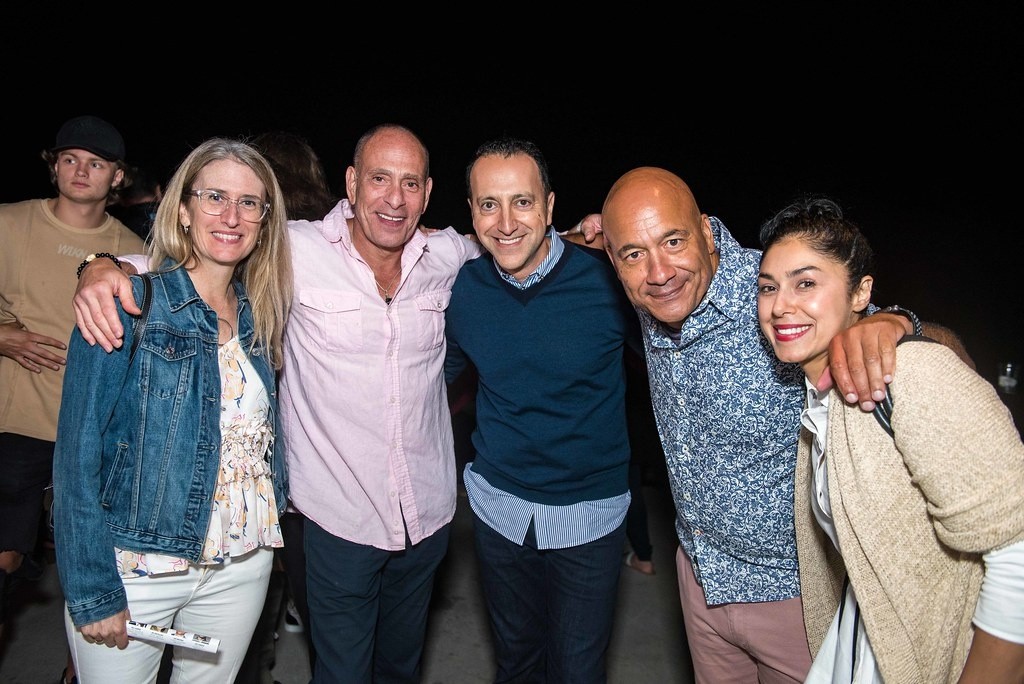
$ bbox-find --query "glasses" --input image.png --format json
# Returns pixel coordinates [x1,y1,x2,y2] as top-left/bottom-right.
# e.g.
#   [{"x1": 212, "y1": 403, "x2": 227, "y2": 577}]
[{"x1": 182, "y1": 187, "x2": 274, "y2": 222}]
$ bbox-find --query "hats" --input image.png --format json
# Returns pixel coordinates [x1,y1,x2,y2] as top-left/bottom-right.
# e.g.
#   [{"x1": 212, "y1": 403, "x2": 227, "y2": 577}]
[{"x1": 51, "y1": 115, "x2": 125, "y2": 161}]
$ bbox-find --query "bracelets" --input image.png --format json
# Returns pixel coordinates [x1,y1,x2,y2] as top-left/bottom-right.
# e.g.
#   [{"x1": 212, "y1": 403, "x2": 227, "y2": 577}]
[
  {"x1": 872, "y1": 305, "x2": 923, "y2": 337},
  {"x1": 77, "y1": 253, "x2": 122, "y2": 279}
]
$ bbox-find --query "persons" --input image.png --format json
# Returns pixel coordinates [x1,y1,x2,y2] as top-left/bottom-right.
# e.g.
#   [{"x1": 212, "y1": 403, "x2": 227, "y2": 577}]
[
  {"x1": 443, "y1": 138, "x2": 632, "y2": 684},
  {"x1": 106, "y1": 149, "x2": 167, "y2": 241},
  {"x1": 250, "y1": 132, "x2": 334, "y2": 220},
  {"x1": 0, "y1": 116, "x2": 148, "y2": 684},
  {"x1": 758, "y1": 201, "x2": 1024, "y2": 684},
  {"x1": 53, "y1": 140, "x2": 293, "y2": 684},
  {"x1": 72, "y1": 124, "x2": 603, "y2": 684},
  {"x1": 622, "y1": 465, "x2": 656, "y2": 572},
  {"x1": 562, "y1": 167, "x2": 975, "y2": 684}
]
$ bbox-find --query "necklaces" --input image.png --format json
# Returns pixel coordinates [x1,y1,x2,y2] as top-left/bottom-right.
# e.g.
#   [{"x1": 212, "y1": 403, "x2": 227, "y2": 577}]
[
  {"x1": 216, "y1": 285, "x2": 233, "y2": 346},
  {"x1": 376, "y1": 269, "x2": 402, "y2": 303}
]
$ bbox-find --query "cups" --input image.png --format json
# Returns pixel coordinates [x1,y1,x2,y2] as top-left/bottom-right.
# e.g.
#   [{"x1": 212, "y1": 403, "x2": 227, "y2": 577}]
[{"x1": 997, "y1": 362, "x2": 1020, "y2": 395}]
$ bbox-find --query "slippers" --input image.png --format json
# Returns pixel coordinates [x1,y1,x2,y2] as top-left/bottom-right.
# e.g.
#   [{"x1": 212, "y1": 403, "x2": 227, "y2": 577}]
[{"x1": 621, "y1": 551, "x2": 655, "y2": 575}]
